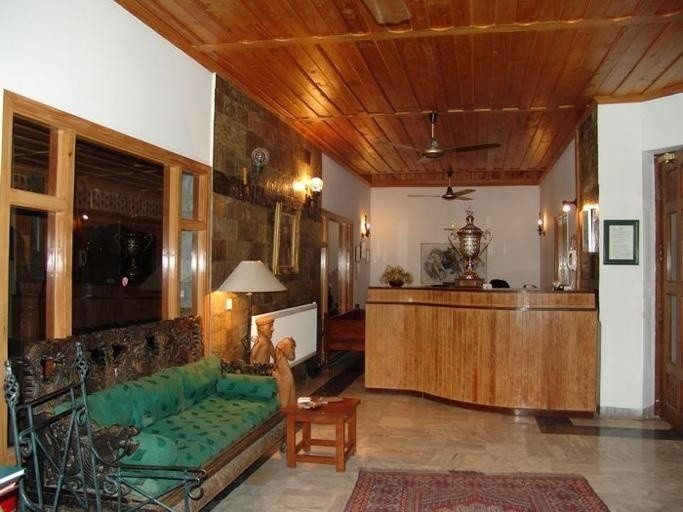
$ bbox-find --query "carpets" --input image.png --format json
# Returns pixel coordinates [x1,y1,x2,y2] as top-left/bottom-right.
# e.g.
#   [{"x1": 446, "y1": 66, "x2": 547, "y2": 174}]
[{"x1": 345, "y1": 471, "x2": 611, "y2": 512}]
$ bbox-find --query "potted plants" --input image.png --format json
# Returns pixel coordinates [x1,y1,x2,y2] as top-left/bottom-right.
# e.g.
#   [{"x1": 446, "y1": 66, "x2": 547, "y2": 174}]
[{"x1": 379, "y1": 264, "x2": 414, "y2": 287}]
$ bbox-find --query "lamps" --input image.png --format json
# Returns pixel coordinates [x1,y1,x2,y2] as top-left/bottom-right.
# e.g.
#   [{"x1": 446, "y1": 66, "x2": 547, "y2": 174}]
[
  {"x1": 561, "y1": 198, "x2": 577, "y2": 212},
  {"x1": 536, "y1": 212, "x2": 546, "y2": 237},
  {"x1": 215, "y1": 260, "x2": 288, "y2": 363},
  {"x1": 305, "y1": 176, "x2": 324, "y2": 208},
  {"x1": 243, "y1": 147, "x2": 270, "y2": 186},
  {"x1": 361, "y1": 215, "x2": 371, "y2": 238}
]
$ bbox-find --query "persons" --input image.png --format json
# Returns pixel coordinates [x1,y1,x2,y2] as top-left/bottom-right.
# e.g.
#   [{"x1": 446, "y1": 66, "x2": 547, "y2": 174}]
[{"x1": 249, "y1": 316, "x2": 276, "y2": 366}]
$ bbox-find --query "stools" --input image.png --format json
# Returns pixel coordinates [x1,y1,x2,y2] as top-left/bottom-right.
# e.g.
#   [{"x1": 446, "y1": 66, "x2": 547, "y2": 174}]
[{"x1": 280, "y1": 395, "x2": 361, "y2": 473}]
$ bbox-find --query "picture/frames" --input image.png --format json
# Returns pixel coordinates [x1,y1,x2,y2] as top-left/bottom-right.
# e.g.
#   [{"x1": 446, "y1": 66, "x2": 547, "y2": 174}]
[
  {"x1": 603, "y1": 219, "x2": 639, "y2": 265},
  {"x1": 272, "y1": 202, "x2": 302, "y2": 277}
]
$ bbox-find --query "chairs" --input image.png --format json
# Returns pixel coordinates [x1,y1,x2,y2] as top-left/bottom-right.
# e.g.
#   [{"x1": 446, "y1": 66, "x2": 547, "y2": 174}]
[
  {"x1": 3, "y1": 341, "x2": 207, "y2": 512},
  {"x1": 490, "y1": 279, "x2": 510, "y2": 288}
]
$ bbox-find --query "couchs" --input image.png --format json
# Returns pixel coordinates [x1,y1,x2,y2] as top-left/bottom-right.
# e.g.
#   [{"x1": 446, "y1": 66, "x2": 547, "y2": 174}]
[{"x1": 23, "y1": 315, "x2": 286, "y2": 512}]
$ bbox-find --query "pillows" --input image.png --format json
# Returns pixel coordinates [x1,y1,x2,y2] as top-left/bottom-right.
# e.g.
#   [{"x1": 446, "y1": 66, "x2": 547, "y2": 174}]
[{"x1": 53, "y1": 353, "x2": 279, "y2": 472}]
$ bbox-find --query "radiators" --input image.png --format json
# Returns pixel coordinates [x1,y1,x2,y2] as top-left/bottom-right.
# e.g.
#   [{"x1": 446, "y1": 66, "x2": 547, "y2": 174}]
[{"x1": 251, "y1": 302, "x2": 317, "y2": 367}]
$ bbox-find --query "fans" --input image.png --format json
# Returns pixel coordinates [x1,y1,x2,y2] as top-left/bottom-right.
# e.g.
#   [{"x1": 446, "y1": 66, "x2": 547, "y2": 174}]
[{"x1": 374, "y1": 110, "x2": 501, "y2": 200}]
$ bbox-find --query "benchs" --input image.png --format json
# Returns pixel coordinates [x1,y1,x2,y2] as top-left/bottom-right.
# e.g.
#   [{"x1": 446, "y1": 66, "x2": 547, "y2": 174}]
[{"x1": 324, "y1": 303, "x2": 365, "y2": 368}]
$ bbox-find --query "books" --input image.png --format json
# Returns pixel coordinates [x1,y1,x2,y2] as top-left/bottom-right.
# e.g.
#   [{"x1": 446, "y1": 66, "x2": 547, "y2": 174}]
[
  {"x1": 0, "y1": 477, "x2": 19, "y2": 496},
  {"x1": 0, "y1": 463, "x2": 26, "y2": 484}
]
